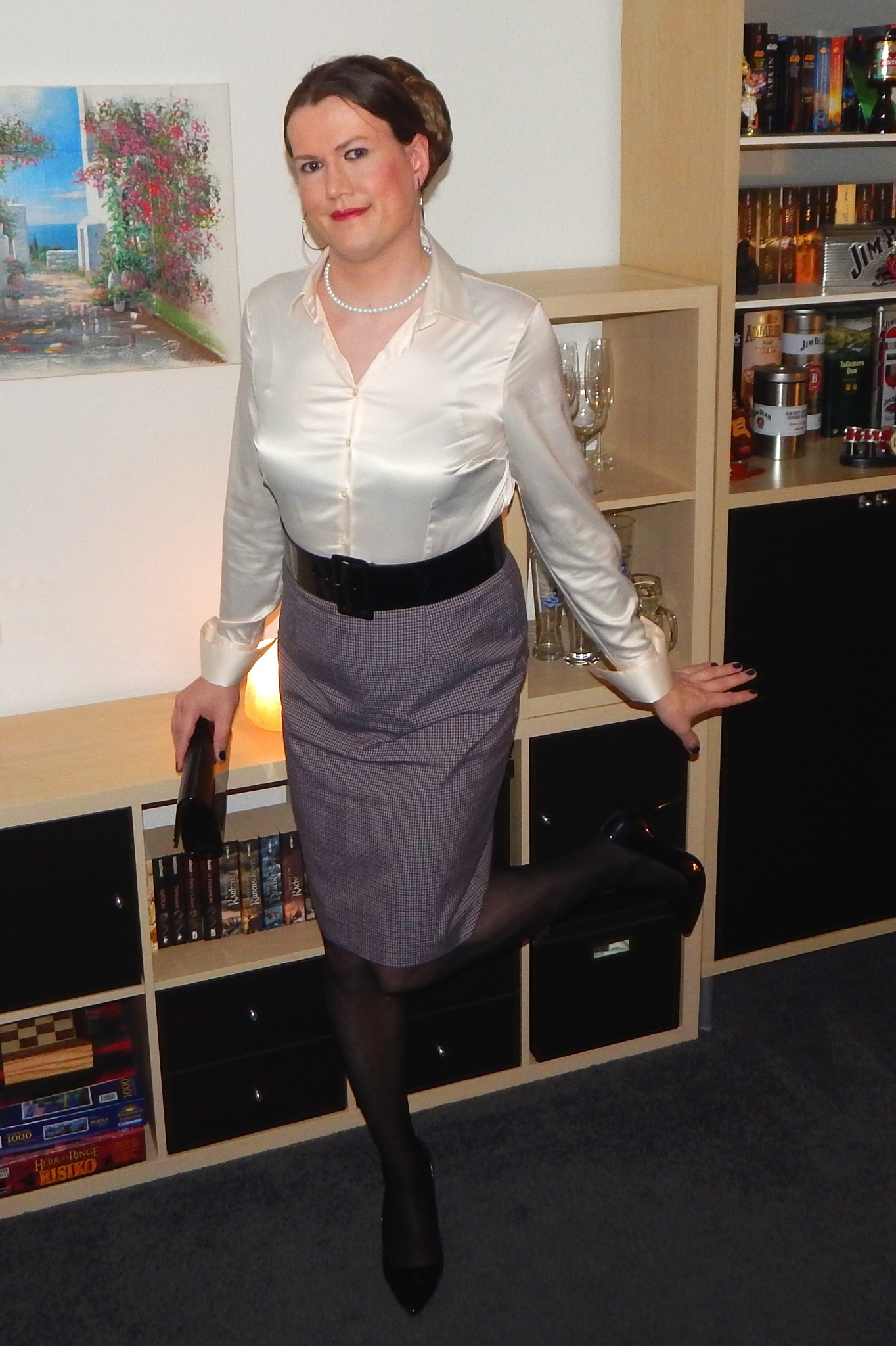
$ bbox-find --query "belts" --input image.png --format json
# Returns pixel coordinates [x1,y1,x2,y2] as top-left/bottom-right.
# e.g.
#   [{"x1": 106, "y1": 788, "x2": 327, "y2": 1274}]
[{"x1": 284, "y1": 516, "x2": 504, "y2": 618}]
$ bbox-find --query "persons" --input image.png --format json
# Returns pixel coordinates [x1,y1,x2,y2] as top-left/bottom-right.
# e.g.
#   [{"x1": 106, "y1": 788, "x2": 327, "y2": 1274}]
[{"x1": 169, "y1": 53, "x2": 757, "y2": 1316}]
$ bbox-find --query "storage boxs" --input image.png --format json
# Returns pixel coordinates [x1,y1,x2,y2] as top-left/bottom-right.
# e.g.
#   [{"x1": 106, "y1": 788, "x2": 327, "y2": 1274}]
[
  {"x1": 734, "y1": 308, "x2": 783, "y2": 415},
  {"x1": 813, "y1": 219, "x2": 896, "y2": 288},
  {"x1": 529, "y1": 916, "x2": 681, "y2": 1062}
]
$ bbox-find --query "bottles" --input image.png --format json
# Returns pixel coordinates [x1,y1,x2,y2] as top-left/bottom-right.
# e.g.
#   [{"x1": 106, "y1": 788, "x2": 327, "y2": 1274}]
[{"x1": 733, "y1": 302, "x2": 896, "y2": 471}]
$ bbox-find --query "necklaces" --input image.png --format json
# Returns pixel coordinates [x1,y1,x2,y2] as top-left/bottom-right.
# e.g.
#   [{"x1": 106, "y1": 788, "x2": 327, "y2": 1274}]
[{"x1": 318, "y1": 254, "x2": 442, "y2": 313}]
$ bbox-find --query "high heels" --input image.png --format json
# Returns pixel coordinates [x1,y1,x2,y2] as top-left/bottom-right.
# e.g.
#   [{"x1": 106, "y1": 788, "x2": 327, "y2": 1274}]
[
  {"x1": 376, "y1": 1160, "x2": 442, "y2": 1317},
  {"x1": 599, "y1": 791, "x2": 704, "y2": 935}
]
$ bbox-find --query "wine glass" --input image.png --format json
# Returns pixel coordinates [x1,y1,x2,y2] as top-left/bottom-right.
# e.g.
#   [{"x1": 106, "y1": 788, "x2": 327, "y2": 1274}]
[{"x1": 561, "y1": 342, "x2": 616, "y2": 479}]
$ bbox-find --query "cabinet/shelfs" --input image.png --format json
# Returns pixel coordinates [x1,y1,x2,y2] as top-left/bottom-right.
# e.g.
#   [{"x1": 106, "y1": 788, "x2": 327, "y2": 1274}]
[{"x1": 1, "y1": 0, "x2": 896, "y2": 1228}]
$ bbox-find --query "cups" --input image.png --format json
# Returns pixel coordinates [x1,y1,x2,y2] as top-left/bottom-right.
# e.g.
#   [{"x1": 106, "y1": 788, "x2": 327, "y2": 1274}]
[{"x1": 529, "y1": 511, "x2": 680, "y2": 668}]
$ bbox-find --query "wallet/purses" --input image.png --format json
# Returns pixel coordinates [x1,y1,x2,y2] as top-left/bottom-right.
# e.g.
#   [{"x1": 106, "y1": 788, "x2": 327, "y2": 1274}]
[{"x1": 169, "y1": 719, "x2": 226, "y2": 862}]
